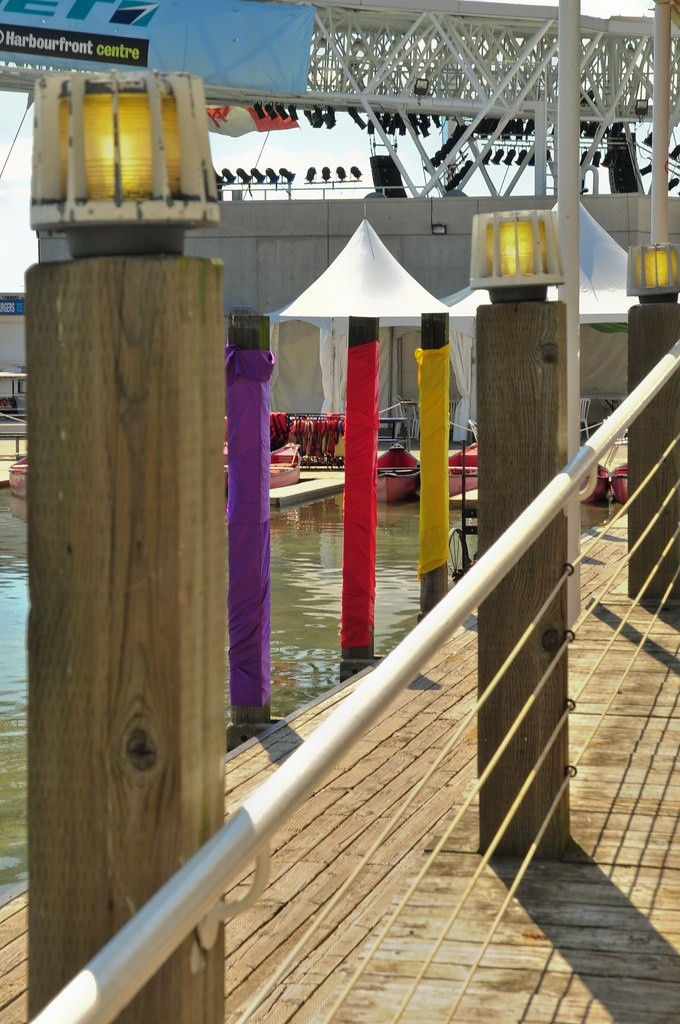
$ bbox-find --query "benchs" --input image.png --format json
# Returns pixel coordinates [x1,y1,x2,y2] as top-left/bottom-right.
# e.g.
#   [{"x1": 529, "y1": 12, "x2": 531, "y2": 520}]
[{"x1": 270, "y1": 466, "x2": 295, "y2": 470}]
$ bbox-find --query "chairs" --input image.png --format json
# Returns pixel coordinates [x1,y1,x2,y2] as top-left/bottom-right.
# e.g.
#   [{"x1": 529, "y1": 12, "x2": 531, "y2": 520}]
[{"x1": 390, "y1": 393, "x2": 630, "y2": 468}]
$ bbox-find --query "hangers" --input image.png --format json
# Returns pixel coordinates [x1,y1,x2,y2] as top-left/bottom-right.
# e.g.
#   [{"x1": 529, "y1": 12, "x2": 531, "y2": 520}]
[{"x1": 288, "y1": 413, "x2": 346, "y2": 429}]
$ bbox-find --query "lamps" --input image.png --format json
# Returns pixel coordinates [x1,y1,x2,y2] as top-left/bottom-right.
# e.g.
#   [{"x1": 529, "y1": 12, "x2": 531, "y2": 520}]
[
  {"x1": 222, "y1": 79, "x2": 680, "y2": 196},
  {"x1": 468, "y1": 210, "x2": 564, "y2": 304},
  {"x1": 28, "y1": 73, "x2": 224, "y2": 258},
  {"x1": 627, "y1": 242, "x2": 680, "y2": 304}
]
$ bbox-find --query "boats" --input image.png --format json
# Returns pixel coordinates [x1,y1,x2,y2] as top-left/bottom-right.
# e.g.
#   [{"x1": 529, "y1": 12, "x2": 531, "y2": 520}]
[
  {"x1": 6, "y1": 457, "x2": 29, "y2": 498},
  {"x1": 223, "y1": 442, "x2": 304, "y2": 492},
  {"x1": 447, "y1": 443, "x2": 481, "y2": 498},
  {"x1": 607, "y1": 457, "x2": 630, "y2": 505},
  {"x1": 374, "y1": 439, "x2": 422, "y2": 502},
  {"x1": 578, "y1": 463, "x2": 610, "y2": 504}
]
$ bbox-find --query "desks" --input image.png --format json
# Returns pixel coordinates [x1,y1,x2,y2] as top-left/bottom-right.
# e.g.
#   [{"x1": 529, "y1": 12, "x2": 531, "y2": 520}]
[
  {"x1": 400, "y1": 398, "x2": 460, "y2": 439},
  {"x1": 580, "y1": 394, "x2": 626, "y2": 414}
]
